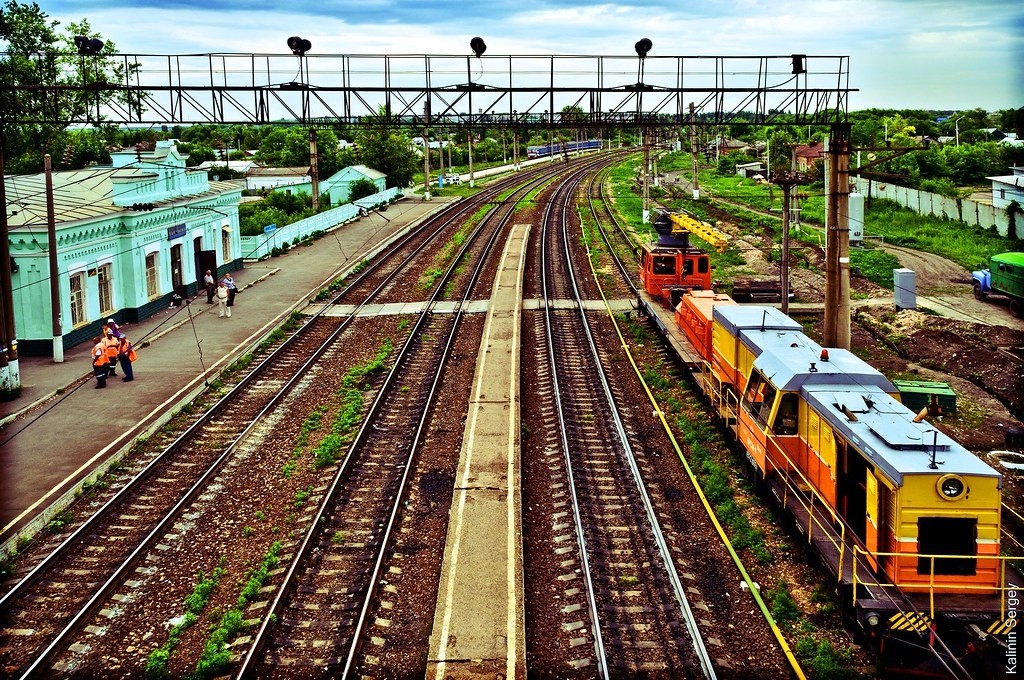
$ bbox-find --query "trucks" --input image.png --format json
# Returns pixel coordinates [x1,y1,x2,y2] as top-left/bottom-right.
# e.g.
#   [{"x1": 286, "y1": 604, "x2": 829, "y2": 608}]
[{"x1": 972, "y1": 252, "x2": 1024, "y2": 317}]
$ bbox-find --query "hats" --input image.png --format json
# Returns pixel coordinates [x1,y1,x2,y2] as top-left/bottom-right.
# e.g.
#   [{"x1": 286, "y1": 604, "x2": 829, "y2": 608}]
[
  {"x1": 119, "y1": 333, "x2": 126, "y2": 339},
  {"x1": 107, "y1": 318, "x2": 115, "y2": 324},
  {"x1": 107, "y1": 329, "x2": 113, "y2": 335}
]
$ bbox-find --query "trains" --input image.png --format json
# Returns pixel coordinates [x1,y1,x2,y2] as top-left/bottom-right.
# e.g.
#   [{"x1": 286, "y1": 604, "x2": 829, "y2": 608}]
[
  {"x1": 527, "y1": 140, "x2": 603, "y2": 160},
  {"x1": 635, "y1": 205, "x2": 1024, "y2": 680}
]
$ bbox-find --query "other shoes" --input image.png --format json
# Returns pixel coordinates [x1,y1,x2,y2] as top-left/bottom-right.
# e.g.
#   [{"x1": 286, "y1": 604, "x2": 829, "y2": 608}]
[
  {"x1": 94, "y1": 382, "x2": 106, "y2": 389},
  {"x1": 218, "y1": 315, "x2": 224, "y2": 318},
  {"x1": 227, "y1": 315, "x2": 231, "y2": 318},
  {"x1": 122, "y1": 377, "x2": 132, "y2": 382},
  {"x1": 109, "y1": 372, "x2": 117, "y2": 376}
]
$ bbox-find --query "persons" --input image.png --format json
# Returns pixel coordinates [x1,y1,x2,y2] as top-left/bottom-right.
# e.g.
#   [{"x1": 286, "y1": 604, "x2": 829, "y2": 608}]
[
  {"x1": 116, "y1": 334, "x2": 136, "y2": 382},
  {"x1": 108, "y1": 319, "x2": 122, "y2": 338},
  {"x1": 92, "y1": 336, "x2": 110, "y2": 389},
  {"x1": 204, "y1": 270, "x2": 216, "y2": 304},
  {"x1": 223, "y1": 273, "x2": 236, "y2": 307},
  {"x1": 101, "y1": 330, "x2": 118, "y2": 378},
  {"x1": 101, "y1": 323, "x2": 112, "y2": 337},
  {"x1": 215, "y1": 282, "x2": 232, "y2": 318}
]
